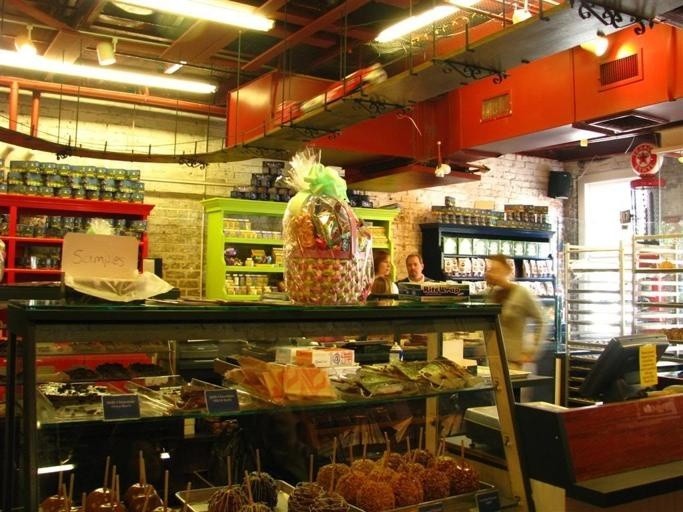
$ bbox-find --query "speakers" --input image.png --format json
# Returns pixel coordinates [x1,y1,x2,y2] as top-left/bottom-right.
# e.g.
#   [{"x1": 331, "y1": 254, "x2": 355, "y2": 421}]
[{"x1": 547, "y1": 171, "x2": 571, "y2": 199}]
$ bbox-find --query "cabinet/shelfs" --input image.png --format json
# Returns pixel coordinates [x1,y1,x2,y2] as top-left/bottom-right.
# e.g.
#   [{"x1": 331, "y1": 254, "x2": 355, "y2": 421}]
[
  {"x1": 564, "y1": 242, "x2": 622, "y2": 348},
  {"x1": 198, "y1": 197, "x2": 400, "y2": 301},
  {"x1": 5, "y1": 299, "x2": 536, "y2": 512},
  {"x1": 419, "y1": 222, "x2": 555, "y2": 347},
  {"x1": 633, "y1": 233, "x2": 682, "y2": 338},
  {"x1": 0, "y1": 193, "x2": 155, "y2": 404}
]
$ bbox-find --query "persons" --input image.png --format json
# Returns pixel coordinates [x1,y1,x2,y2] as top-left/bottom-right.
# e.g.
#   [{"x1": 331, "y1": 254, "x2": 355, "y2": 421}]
[
  {"x1": 394, "y1": 254, "x2": 439, "y2": 288},
  {"x1": 481, "y1": 254, "x2": 544, "y2": 371},
  {"x1": 365, "y1": 250, "x2": 399, "y2": 344}
]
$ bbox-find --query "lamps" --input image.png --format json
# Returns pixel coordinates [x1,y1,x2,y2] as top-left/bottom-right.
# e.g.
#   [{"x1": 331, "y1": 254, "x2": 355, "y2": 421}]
[
  {"x1": 0, "y1": 11, "x2": 170, "y2": 66},
  {"x1": 580, "y1": 37, "x2": 607, "y2": 56},
  {"x1": 435, "y1": 142, "x2": 451, "y2": 178}
]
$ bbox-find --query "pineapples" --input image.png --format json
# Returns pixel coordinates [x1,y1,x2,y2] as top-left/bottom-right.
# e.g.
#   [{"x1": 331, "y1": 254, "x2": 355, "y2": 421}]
[{"x1": 276, "y1": 150, "x2": 375, "y2": 307}]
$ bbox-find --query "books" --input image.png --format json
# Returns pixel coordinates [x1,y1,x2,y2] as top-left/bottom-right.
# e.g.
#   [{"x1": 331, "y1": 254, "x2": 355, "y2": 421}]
[{"x1": 398, "y1": 280, "x2": 469, "y2": 305}]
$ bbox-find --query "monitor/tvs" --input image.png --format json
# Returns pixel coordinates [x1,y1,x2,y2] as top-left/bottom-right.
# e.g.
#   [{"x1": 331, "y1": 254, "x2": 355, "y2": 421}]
[{"x1": 579, "y1": 333, "x2": 668, "y2": 402}]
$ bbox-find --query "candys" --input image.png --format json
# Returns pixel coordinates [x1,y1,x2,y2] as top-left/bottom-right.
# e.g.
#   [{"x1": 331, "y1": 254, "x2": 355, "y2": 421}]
[{"x1": 41, "y1": 448, "x2": 479, "y2": 512}]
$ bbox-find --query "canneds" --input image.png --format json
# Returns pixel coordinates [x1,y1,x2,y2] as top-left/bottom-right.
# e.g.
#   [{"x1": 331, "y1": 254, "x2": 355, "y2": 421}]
[
  {"x1": 0, "y1": 157, "x2": 147, "y2": 273},
  {"x1": 230, "y1": 161, "x2": 372, "y2": 207},
  {"x1": 223, "y1": 219, "x2": 389, "y2": 295},
  {"x1": 431, "y1": 196, "x2": 551, "y2": 230}
]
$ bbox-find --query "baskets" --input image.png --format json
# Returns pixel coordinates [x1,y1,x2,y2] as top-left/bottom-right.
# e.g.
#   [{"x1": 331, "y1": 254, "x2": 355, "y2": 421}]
[{"x1": 282, "y1": 202, "x2": 372, "y2": 305}]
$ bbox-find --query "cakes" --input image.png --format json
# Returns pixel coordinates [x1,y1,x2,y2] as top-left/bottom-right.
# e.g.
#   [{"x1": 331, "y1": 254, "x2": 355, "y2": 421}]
[{"x1": 38, "y1": 381, "x2": 218, "y2": 420}]
